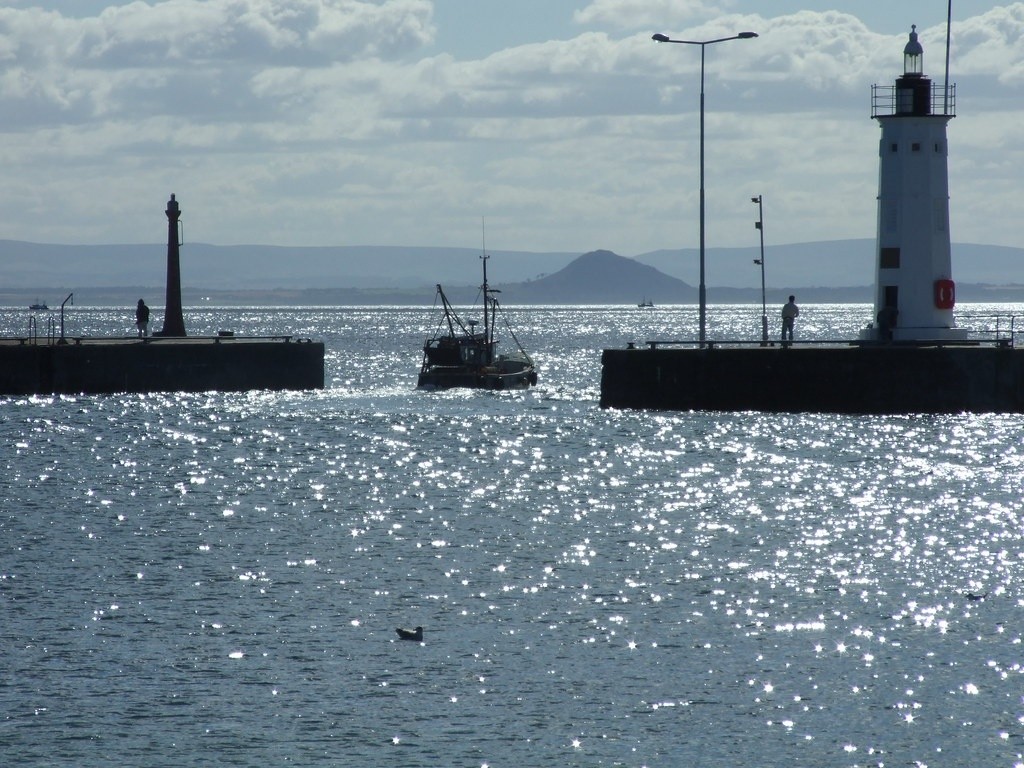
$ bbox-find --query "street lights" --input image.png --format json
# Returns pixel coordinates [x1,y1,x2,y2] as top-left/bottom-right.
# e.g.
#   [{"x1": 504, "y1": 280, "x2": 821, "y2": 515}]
[{"x1": 652, "y1": 32, "x2": 760, "y2": 348}]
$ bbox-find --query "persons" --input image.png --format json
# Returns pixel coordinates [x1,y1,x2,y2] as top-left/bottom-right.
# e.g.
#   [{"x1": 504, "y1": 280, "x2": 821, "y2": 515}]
[
  {"x1": 136, "y1": 299, "x2": 149, "y2": 337},
  {"x1": 781, "y1": 295, "x2": 799, "y2": 348}
]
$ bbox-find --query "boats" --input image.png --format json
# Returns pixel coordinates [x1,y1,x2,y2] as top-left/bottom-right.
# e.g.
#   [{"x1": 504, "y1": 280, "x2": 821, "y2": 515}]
[
  {"x1": 417, "y1": 216, "x2": 537, "y2": 392},
  {"x1": 29, "y1": 297, "x2": 49, "y2": 309},
  {"x1": 638, "y1": 296, "x2": 654, "y2": 307}
]
